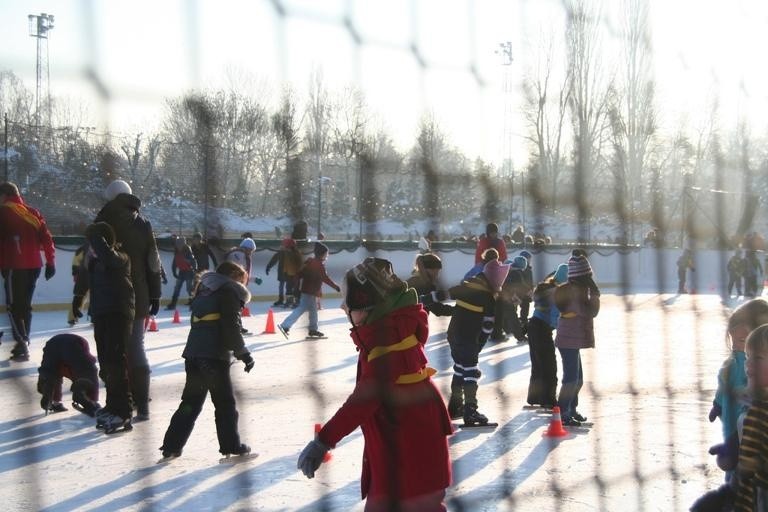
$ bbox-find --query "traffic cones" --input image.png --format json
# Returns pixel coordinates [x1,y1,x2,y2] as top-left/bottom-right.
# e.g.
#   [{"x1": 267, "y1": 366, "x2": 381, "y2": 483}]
[
  {"x1": 147, "y1": 314, "x2": 159, "y2": 332},
  {"x1": 172, "y1": 309, "x2": 181, "y2": 323},
  {"x1": 542, "y1": 406, "x2": 572, "y2": 439},
  {"x1": 171, "y1": 309, "x2": 182, "y2": 325},
  {"x1": 543, "y1": 406, "x2": 566, "y2": 437},
  {"x1": 241, "y1": 302, "x2": 251, "y2": 318},
  {"x1": 263, "y1": 309, "x2": 276, "y2": 334},
  {"x1": 262, "y1": 307, "x2": 277, "y2": 335},
  {"x1": 148, "y1": 319, "x2": 160, "y2": 331}
]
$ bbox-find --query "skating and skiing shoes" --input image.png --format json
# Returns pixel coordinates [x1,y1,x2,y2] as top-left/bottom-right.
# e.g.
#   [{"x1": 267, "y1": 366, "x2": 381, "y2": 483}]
[
  {"x1": 517, "y1": 338, "x2": 530, "y2": 343},
  {"x1": 573, "y1": 411, "x2": 587, "y2": 421},
  {"x1": 46, "y1": 400, "x2": 68, "y2": 410},
  {"x1": 165, "y1": 304, "x2": 176, "y2": 310},
  {"x1": 72, "y1": 388, "x2": 100, "y2": 408},
  {"x1": 529, "y1": 398, "x2": 543, "y2": 406},
  {"x1": 98, "y1": 418, "x2": 133, "y2": 434},
  {"x1": 24, "y1": 336, "x2": 31, "y2": 345},
  {"x1": 11, "y1": 342, "x2": 27, "y2": 352},
  {"x1": 541, "y1": 401, "x2": 558, "y2": 408},
  {"x1": 219, "y1": 443, "x2": 259, "y2": 464},
  {"x1": 449, "y1": 411, "x2": 465, "y2": 417},
  {"x1": 561, "y1": 416, "x2": 580, "y2": 425},
  {"x1": 278, "y1": 323, "x2": 290, "y2": 339},
  {"x1": 489, "y1": 336, "x2": 508, "y2": 342},
  {"x1": 464, "y1": 412, "x2": 488, "y2": 424},
  {"x1": 281, "y1": 304, "x2": 292, "y2": 307},
  {"x1": 15, "y1": 349, "x2": 19, "y2": 349},
  {"x1": 274, "y1": 301, "x2": 283, "y2": 305},
  {"x1": 158, "y1": 440, "x2": 184, "y2": 457},
  {"x1": 305, "y1": 330, "x2": 327, "y2": 340},
  {"x1": 184, "y1": 300, "x2": 193, "y2": 306},
  {"x1": 95, "y1": 413, "x2": 113, "y2": 423}
]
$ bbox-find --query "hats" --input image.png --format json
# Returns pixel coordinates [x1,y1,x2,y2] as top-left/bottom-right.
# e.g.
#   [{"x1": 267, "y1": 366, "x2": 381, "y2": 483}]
[
  {"x1": 342, "y1": 257, "x2": 407, "y2": 311},
  {"x1": 240, "y1": 238, "x2": 256, "y2": 254},
  {"x1": 554, "y1": 264, "x2": 569, "y2": 284},
  {"x1": 96, "y1": 179, "x2": 143, "y2": 216},
  {"x1": 284, "y1": 238, "x2": 296, "y2": 248},
  {"x1": 515, "y1": 255, "x2": 527, "y2": 271},
  {"x1": 484, "y1": 248, "x2": 500, "y2": 261},
  {"x1": 520, "y1": 251, "x2": 532, "y2": 264},
  {"x1": 192, "y1": 231, "x2": 202, "y2": 240},
  {"x1": 485, "y1": 259, "x2": 511, "y2": 290},
  {"x1": 568, "y1": 249, "x2": 593, "y2": 278},
  {"x1": 177, "y1": 237, "x2": 186, "y2": 246},
  {"x1": 486, "y1": 223, "x2": 498, "y2": 233},
  {"x1": 314, "y1": 243, "x2": 328, "y2": 255},
  {"x1": 418, "y1": 254, "x2": 442, "y2": 271},
  {"x1": 86, "y1": 222, "x2": 115, "y2": 254}
]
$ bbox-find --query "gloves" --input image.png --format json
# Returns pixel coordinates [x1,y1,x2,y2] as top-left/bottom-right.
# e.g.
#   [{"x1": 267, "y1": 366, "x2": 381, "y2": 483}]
[
  {"x1": 708, "y1": 434, "x2": 738, "y2": 469},
  {"x1": 709, "y1": 402, "x2": 722, "y2": 422},
  {"x1": 335, "y1": 286, "x2": 341, "y2": 292},
  {"x1": 73, "y1": 275, "x2": 80, "y2": 282},
  {"x1": 45, "y1": 266, "x2": 55, "y2": 281},
  {"x1": 266, "y1": 267, "x2": 270, "y2": 276},
  {"x1": 151, "y1": 298, "x2": 161, "y2": 317},
  {"x1": 173, "y1": 271, "x2": 180, "y2": 279},
  {"x1": 163, "y1": 275, "x2": 168, "y2": 285},
  {"x1": 256, "y1": 277, "x2": 262, "y2": 285},
  {"x1": 72, "y1": 296, "x2": 83, "y2": 319},
  {"x1": 691, "y1": 268, "x2": 696, "y2": 272},
  {"x1": 96, "y1": 221, "x2": 107, "y2": 236},
  {"x1": 296, "y1": 439, "x2": 325, "y2": 477},
  {"x1": 40, "y1": 396, "x2": 50, "y2": 410},
  {"x1": 590, "y1": 281, "x2": 598, "y2": 291},
  {"x1": 241, "y1": 352, "x2": 255, "y2": 372}
]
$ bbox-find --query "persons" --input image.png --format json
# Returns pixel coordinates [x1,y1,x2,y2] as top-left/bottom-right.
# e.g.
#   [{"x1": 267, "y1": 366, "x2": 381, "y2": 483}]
[
  {"x1": 93, "y1": 179, "x2": 162, "y2": 424},
  {"x1": 517, "y1": 251, "x2": 533, "y2": 334},
  {"x1": 283, "y1": 239, "x2": 303, "y2": 309},
  {"x1": 164, "y1": 236, "x2": 198, "y2": 312},
  {"x1": 277, "y1": 242, "x2": 342, "y2": 340},
  {"x1": 709, "y1": 299, "x2": 768, "y2": 483},
  {"x1": 676, "y1": 248, "x2": 696, "y2": 294},
  {"x1": 689, "y1": 324, "x2": 768, "y2": 512},
  {"x1": 297, "y1": 255, "x2": 456, "y2": 512},
  {"x1": 190, "y1": 234, "x2": 218, "y2": 273},
  {"x1": 157, "y1": 260, "x2": 259, "y2": 467},
  {"x1": 0, "y1": 182, "x2": 57, "y2": 362},
  {"x1": 418, "y1": 260, "x2": 511, "y2": 428},
  {"x1": 225, "y1": 237, "x2": 262, "y2": 334},
  {"x1": 403, "y1": 253, "x2": 457, "y2": 320},
  {"x1": 726, "y1": 232, "x2": 767, "y2": 298},
  {"x1": 460, "y1": 248, "x2": 499, "y2": 284},
  {"x1": 554, "y1": 249, "x2": 600, "y2": 429},
  {"x1": 474, "y1": 222, "x2": 508, "y2": 266},
  {"x1": 524, "y1": 270, "x2": 561, "y2": 415},
  {"x1": 502, "y1": 225, "x2": 553, "y2": 245},
  {"x1": 37, "y1": 333, "x2": 103, "y2": 418},
  {"x1": 266, "y1": 239, "x2": 290, "y2": 309},
  {"x1": 71, "y1": 222, "x2": 136, "y2": 436},
  {"x1": 67, "y1": 243, "x2": 96, "y2": 328},
  {"x1": 492, "y1": 256, "x2": 528, "y2": 343},
  {"x1": 149, "y1": 261, "x2": 169, "y2": 319}
]
